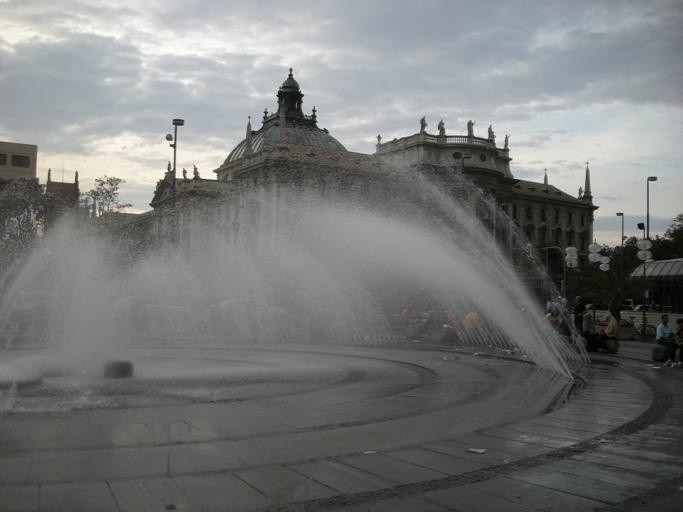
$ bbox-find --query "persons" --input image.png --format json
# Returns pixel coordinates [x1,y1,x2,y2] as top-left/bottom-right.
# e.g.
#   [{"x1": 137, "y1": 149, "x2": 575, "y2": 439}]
[
  {"x1": 402, "y1": 290, "x2": 635, "y2": 354},
  {"x1": 661, "y1": 317, "x2": 682, "y2": 370},
  {"x1": 656, "y1": 314, "x2": 675, "y2": 362}
]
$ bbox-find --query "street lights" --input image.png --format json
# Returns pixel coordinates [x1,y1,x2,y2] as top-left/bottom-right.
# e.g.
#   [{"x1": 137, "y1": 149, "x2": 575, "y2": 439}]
[
  {"x1": 635, "y1": 222, "x2": 649, "y2": 338},
  {"x1": 168, "y1": 118, "x2": 185, "y2": 194},
  {"x1": 615, "y1": 211, "x2": 624, "y2": 286},
  {"x1": 645, "y1": 175, "x2": 658, "y2": 239}
]
